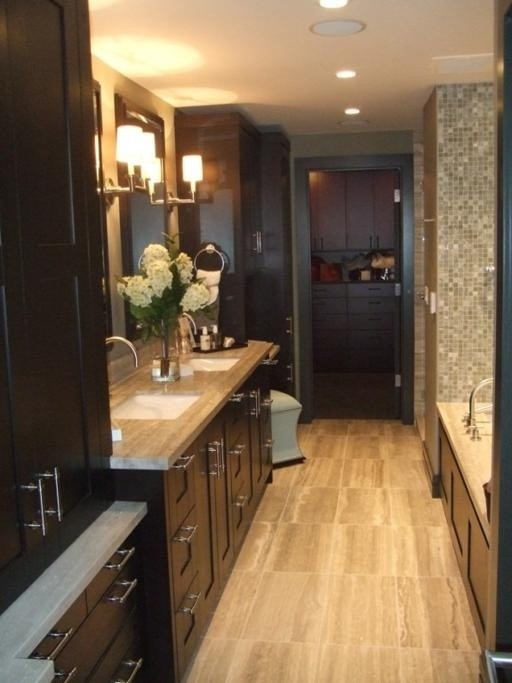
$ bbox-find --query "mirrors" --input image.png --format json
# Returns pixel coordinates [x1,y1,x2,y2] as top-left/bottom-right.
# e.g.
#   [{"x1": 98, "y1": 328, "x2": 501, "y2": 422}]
[{"x1": 114, "y1": 93, "x2": 171, "y2": 341}]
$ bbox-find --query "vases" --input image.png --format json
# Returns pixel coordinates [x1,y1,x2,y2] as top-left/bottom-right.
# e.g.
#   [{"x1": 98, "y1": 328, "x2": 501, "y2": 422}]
[{"x1": 151, "y1": 318, "x2": 180, "y2": 382}]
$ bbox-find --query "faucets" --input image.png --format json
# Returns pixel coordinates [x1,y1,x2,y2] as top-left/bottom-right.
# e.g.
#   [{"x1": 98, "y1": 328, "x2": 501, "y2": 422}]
[
  {"x1": 468, "y1": 376, "x2": 493, "y2": 437},
  {"x1": 105, "y1": 334, "x2": 139, "y2": 370},
  {"x1": 181, "y1": 311, "x2": 198, "y2": 337}
]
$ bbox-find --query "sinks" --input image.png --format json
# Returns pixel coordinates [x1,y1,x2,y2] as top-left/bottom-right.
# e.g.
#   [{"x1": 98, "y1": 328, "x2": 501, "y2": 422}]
[
  {"x1": 110, "y1": 391, "x2": 200, "y2": 421},
  {"x1": 188, "y1": 356, "x2": 240, "y2": 371}
]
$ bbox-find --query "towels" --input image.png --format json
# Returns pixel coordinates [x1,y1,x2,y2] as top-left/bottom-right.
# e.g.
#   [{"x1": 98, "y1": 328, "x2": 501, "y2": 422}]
[{"x1": 196, "y1": 269, "x2": 221, "y2": 309}]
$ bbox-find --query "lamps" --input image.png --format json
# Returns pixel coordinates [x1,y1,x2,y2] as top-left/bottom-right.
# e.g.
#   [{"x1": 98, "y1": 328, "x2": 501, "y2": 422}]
[{"x1": 103, "y1": 125, "x2": 203, "y2": 214}]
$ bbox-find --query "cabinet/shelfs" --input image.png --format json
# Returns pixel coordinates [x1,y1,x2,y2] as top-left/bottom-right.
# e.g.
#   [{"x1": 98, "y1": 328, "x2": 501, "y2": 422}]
[
  {"x1": 312, "y1": 283, "x2": 393, "y2": 372},
  {"x1": 309, "y1": 172, "x2": 394, "y2": 251},
  {"x1": 176, "y1": 113, "x2": 295, "y2": 397}
]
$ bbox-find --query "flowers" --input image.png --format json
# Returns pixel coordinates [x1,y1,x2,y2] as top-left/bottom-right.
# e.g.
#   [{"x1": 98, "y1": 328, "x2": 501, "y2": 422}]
[{"x1": 114, "y1": 230, "x2": 214, "y2": 376}]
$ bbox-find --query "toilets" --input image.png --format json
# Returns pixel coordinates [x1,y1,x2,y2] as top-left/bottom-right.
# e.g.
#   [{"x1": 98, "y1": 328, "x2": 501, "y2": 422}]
[{"x1": 269, "y1": 388, "x2": 306, "y2": 466}]
